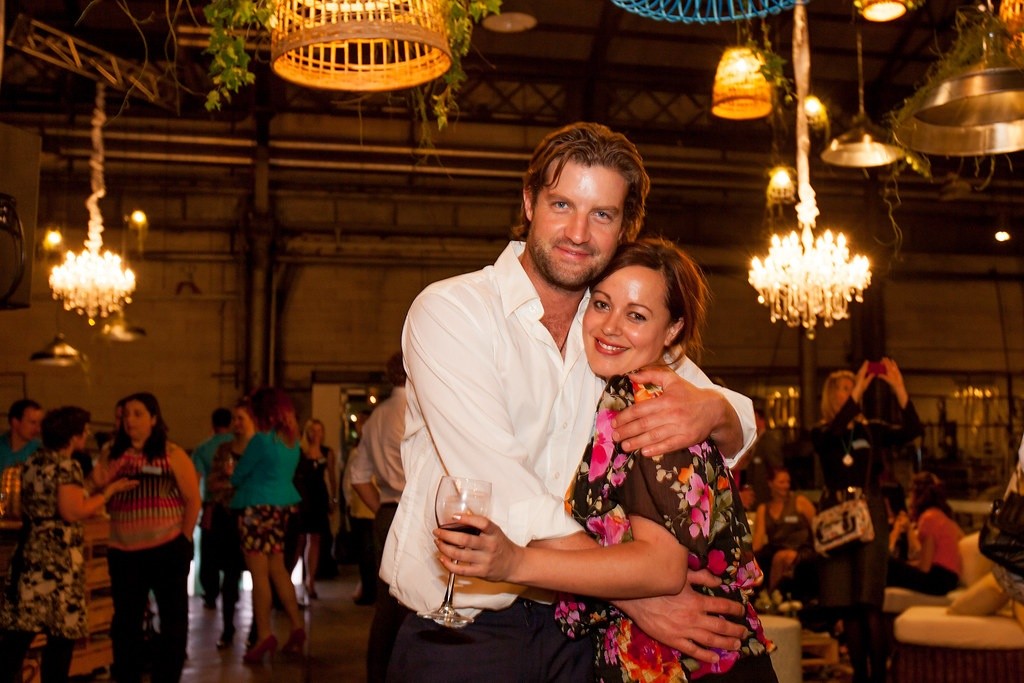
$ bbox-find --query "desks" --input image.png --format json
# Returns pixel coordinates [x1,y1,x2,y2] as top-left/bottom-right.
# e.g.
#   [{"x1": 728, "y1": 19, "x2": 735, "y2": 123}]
[{"x1": 801, "y1": 638, "x2": 838, "y2": 665}]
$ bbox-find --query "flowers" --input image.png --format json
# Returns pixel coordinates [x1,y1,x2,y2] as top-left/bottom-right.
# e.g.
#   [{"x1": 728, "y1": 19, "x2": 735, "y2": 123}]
[{"x1": 754, "y1": 590, "x2": 802, "y2": 616}]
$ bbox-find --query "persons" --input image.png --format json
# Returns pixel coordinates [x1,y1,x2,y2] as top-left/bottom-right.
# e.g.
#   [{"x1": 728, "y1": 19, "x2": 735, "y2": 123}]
[
  {"x1": 987, "y1": 430, "x2": 1024, "y2": 607},
  {"x1": 92, "y1": 394, "x2": 201, "y2": 683},
  {"x1": 752, "y1": 466, "x2": 818, "y2": 605},
  {"x1": 366, "y1": 123, "x2": 757, "y2": 683},
  {"x1": 810, "y1": 358, "x2": 924, "y2": 683},
  {"x1": 0, "y1": 399, "x2": 47, "y2": 598},
  {"x1": 223, "y1": 387, "x2": 305, "y2": 662},
  {"x1": 350, "y1": 350, "x2": 408, "y2": 607},
  {"x1": 208, "y1": 398, "x2": 272, "y2": 649},
  {"x1": 190, "y1": 407, "x2": 243, "y2": 614},
  {"x1": 0, "y1": 406, "x2": 140, "y2": 683},
  {"x1": 342, "y1": 398, "x2": 385, "y2": 604},
  {"x1": 887, "y1": 472, "x2": 967, "y2": 595},
  {"x1": 737, "y1": 482, "x2": 772, "y2": 600},
  {"x1": 269, "y1": 407, "x2": 339, "y2": 612},
  {"x1": 432, "y1": 237, "x2": 778, "y2": 683}
]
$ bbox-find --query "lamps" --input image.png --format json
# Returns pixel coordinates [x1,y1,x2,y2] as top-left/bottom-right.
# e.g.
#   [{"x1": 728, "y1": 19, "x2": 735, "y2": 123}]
[
  {"x1": 103, "y1": 229, "x2": 146, "y2": 342},
  {"x1": 713, "y1": 0, "x2": 1024, "y2": 340},
  {"x1": 49, "y1": 82, "x2": 137, "y2": 325},
  {"x1": 30, "y1": 300, "x2": 78, "y2": 366},
  {"x1": 482, "y1": 0, "x2": 537, "y2": 33}
]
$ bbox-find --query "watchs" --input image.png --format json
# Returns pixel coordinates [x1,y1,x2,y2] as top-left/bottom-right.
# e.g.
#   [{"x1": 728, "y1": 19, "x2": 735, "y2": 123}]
[{"x1": 329, "y1": 496, "x2": 339, "y2": 503}]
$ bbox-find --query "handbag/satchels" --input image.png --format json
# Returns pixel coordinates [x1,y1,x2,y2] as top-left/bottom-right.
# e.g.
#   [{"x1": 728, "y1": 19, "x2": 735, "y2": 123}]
[
  {"x1": 811, "y1": 498, "x2": 874, "y2": 557},
  {"x1": 977, "y1": 491, "x2": 1024, "y2": 578}
]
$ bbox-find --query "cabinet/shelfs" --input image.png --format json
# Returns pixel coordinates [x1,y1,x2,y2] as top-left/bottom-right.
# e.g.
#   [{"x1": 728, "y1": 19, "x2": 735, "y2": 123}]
[{"x1": 0, "y1": 519, "x2": 115, "y2": 683}]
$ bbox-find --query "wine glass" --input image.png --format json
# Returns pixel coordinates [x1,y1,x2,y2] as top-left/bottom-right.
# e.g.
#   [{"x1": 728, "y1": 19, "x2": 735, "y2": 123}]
[{"x1": 415, "y1": 476, "x2": 494, "y2": 622}]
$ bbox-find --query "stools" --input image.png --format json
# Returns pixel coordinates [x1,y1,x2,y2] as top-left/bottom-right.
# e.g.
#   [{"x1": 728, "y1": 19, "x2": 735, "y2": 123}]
[{"x1": 757, "y1": 615, "x2": 802, "y2": 683}]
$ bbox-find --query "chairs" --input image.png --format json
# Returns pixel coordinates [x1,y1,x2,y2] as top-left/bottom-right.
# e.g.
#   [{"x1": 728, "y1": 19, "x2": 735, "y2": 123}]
[{"x1": 884, "y1": 530, "x2": 1024, "y2": 683}]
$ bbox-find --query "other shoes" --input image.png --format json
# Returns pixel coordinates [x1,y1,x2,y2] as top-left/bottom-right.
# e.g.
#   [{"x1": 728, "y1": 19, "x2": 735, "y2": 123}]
[{"x1": 204, "y1": 603, "x2": 216, "y2": 611}]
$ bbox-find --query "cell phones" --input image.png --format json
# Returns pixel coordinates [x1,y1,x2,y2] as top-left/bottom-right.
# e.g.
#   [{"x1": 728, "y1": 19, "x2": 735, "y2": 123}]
[{"x1": 867, "y1": 362, "x2": 887, "y2": 375}]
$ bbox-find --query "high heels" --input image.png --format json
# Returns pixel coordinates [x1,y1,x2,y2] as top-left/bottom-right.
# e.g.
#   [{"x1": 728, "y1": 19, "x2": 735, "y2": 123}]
[
  {"x1": 281, "y1": 628, "x2": 305, "y2": 660},
  {"x1": 242, "y1": 634, "x2": 279, "y2": 667},
  {"x1": 305, "y1": 584, "x2": 318, "y2": 601},
  {"x1": 216, "y1": 627, "x2": 236, "y2": 648}
]
